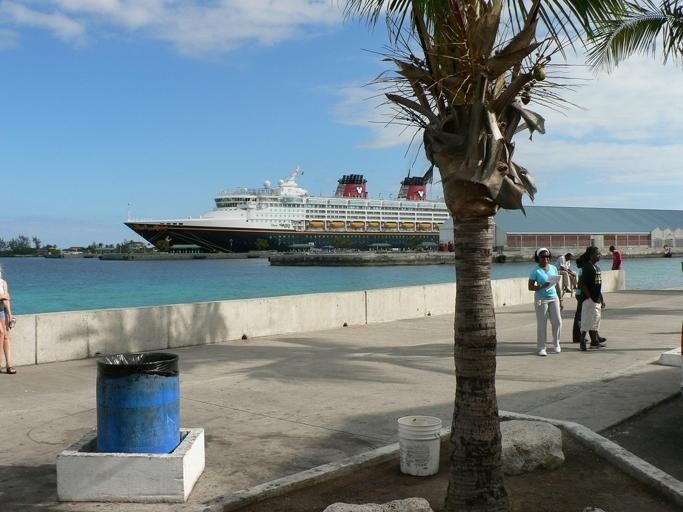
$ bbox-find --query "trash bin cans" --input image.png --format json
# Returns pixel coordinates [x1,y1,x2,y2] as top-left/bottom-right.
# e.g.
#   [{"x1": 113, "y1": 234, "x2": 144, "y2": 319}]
[{"x1": 96, "y1": 352, "x2": 180, "y2": 454}]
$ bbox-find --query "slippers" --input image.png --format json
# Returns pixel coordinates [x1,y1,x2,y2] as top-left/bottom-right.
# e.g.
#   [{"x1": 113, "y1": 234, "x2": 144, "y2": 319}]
[{"x1": 7, "y1": 367, "x2": 16, "y2": 374}]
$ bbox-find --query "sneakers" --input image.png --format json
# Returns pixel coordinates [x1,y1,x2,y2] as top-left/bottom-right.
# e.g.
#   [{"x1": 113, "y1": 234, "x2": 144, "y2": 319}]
[
  {"x1": 566, "y1": 288, "x2": 574, "y2": 292},
  {"x1": 554, "y1": 343, "x2": 561, "y2": 353},
  {"x1": 574, "y1": 337, "x2": 606, "y2": 350},
  {"x1": 538, "y1": 349, "x2": 547, "y2": 356}
]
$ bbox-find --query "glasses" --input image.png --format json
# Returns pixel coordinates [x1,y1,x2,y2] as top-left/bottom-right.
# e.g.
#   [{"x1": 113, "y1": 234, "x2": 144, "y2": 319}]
[{"x1": 541, "y1": 255, "x2": 549, "y2": 258}]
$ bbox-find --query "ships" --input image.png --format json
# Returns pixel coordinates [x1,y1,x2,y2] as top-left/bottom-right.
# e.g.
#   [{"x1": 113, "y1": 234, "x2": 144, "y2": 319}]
[{"x1": 123, "y1": 166, "x2": 450, "y2": 253}]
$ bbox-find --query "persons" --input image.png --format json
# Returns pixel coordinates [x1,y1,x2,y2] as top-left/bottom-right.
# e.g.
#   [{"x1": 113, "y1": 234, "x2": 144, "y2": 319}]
[
  {"x1": 527, "y1": 247, "x2": 564, "y2": 356},
  {"x1": 572, "y1": 246, "x2": 607, "y2": 351},
  {"x1": 609, "y1": 246, "x2": 622, "y2": 269},
  {"x1": 554, "y1": 252, "x2": 578, "y2": 294},
  {"x1": 0, "y1": 267, "x2": 17, "y2": 374}
]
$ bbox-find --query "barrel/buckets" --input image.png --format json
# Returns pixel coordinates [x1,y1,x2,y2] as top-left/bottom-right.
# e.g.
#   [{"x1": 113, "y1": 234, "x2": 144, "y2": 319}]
[{"x1": 397, "y1": 416, "x2": 442, "y2": 476}]
